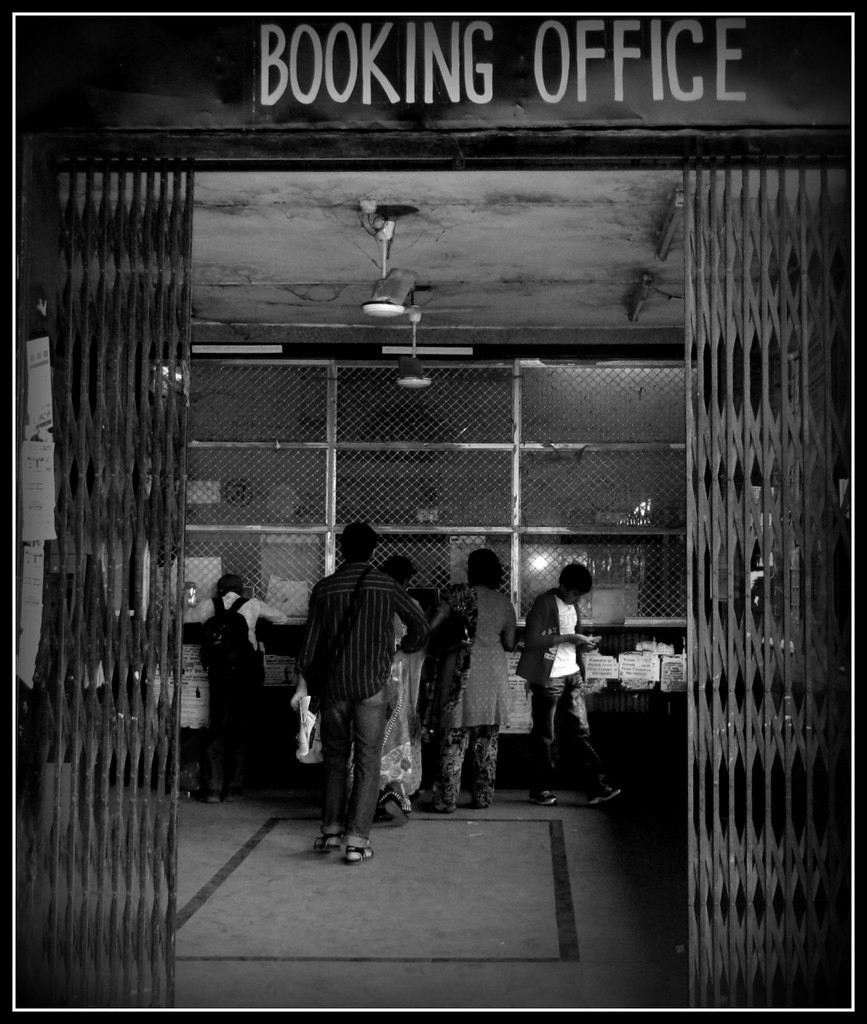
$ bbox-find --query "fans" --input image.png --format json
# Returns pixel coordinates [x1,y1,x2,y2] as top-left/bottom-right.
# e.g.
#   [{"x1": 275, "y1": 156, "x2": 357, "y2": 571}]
[{"x1": 306, "y1": 206, "x2": 528, "y2": 397}]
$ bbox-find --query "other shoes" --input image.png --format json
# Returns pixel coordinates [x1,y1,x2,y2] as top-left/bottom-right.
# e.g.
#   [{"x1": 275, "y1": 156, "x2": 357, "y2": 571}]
[
  {"x1": 384, "y1": 799, "x2": 409, "y2": 824},
  {"x1": 204, "y1": 789, "x2": 221, "y2": 804}
]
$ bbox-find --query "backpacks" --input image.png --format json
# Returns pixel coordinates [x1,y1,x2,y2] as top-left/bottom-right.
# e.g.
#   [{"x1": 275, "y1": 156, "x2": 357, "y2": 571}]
[{"x1": 198, "y1": 594, "x2": 249, "y2": 680}]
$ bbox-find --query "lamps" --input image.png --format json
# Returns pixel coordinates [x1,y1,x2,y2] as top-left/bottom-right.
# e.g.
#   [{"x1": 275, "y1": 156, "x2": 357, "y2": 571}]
[
  {"x1": 656, "y1": 187, "x2": 687, "y2": 263},
  {"x1": 627, "y1": 277, "x2": 654, "y2": 324}
]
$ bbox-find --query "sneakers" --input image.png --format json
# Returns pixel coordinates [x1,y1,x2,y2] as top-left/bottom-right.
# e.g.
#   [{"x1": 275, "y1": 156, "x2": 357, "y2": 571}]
[
  {"x1": 529, "y1": 789, "x2": 558, "y2": 806},
  {"x1": 345, "y1": 845, "x2": 374, "y2": 865},
  {"x1": 314, "y1": 835, "x2": 341, "y2": 854},
  {"x1": 585, "y1": 782, "x2": 622, "y2": 805}
]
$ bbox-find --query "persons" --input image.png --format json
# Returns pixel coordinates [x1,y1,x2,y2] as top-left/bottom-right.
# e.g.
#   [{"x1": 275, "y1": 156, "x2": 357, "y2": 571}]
[
  {"x1": 424, "y1": 549, "x2": 518, "y2": 812},
  {"x1": 290, "y1": 523, "x2": 429, "y2": 864},
  {"x1": 376, "y1": 556, "x2": 428, "y2": 824},
  {"x1": 514, "y1": 563, "x2": 621, "y2": 806},
  {"x1": 183, "y1": 574, "x2": 286, "y2": 804}
]
